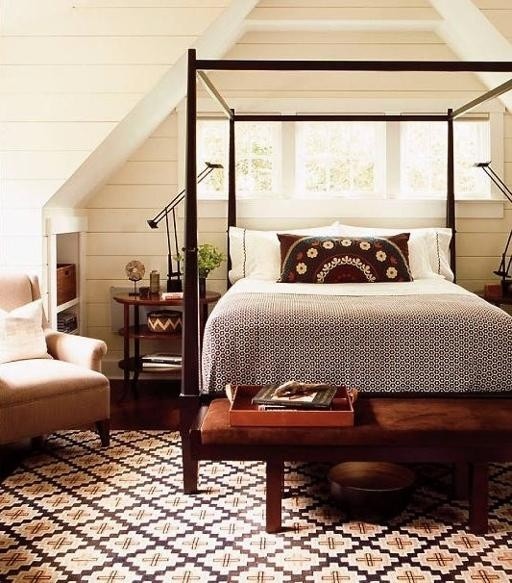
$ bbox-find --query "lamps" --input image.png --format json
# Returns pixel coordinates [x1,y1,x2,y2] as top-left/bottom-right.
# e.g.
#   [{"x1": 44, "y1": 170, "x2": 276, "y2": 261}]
[
  {"x1": 470, "y1": 157, "x2": 512, "y2": 299},
  {"x1": 147, "y1": 159, "x2": 225, "y2": 292}
]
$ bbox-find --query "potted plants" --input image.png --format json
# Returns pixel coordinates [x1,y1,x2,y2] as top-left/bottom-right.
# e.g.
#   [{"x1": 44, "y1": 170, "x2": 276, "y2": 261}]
[{"x1": 172, "y1": 241, "x2": 228, "y2": 296}]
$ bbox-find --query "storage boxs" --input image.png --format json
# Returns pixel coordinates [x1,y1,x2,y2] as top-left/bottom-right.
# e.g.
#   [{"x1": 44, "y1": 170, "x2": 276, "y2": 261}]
[{"x1": 56, "y1": 263, "x2": 76, "y2": 308}]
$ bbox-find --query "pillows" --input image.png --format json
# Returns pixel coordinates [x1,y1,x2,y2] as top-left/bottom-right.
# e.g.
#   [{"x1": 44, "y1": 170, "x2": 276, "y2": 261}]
[
  {"x1": 227, "y1": 222, "x2": 334, "y2": 285},
  {"x1": 0, "y1": 296, "x2": 57, "y2": 363},
  {"x1": 276, "y1": 230, "x2": 414, "y2": 283},
  {"x1": 334, "y1": 220, "x2": 457, "y2": 283}
]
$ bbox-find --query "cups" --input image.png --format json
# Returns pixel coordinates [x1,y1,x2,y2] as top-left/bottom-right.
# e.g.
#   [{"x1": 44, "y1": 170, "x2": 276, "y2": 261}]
[{"x1": 485, "y1": 284, "x2": 501, "y2": 301}]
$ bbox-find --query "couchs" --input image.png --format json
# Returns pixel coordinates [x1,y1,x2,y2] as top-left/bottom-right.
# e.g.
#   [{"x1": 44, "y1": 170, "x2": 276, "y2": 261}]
[{"x1": 0, "y1": 273, "x2": 114, "y2": 450}]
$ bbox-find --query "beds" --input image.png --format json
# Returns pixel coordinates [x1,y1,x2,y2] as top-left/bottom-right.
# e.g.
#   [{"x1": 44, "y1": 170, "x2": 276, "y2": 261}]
[{"x1": 180, "y1": 46, "x2": 512, "y2": 494}]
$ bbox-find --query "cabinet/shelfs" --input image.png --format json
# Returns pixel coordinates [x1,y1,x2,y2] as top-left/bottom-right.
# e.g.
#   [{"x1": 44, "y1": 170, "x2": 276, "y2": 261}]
[{"x1": 47, "y1": 219, "x2": 87, "y2": 338}]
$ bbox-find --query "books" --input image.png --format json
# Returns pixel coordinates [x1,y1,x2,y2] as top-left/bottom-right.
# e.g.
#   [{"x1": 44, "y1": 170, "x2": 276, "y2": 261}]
[
  {"x1": 58, "y1": 310, "x2": 77, "y2": 332},
  {"x1": 138, "y1": 352, "x2": 182, "y2": 373},
  {"x1": 162, "y1": 291, "x2": 183, "y2": 300},
  {"x1": 251, "y1": 380, "x2": 338, "y2": 413}
]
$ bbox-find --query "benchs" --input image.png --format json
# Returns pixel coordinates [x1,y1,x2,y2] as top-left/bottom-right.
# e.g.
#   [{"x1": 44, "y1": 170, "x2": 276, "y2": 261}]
[{"x1": 188, "y1": 395, "x2": 512, "y2": 536}]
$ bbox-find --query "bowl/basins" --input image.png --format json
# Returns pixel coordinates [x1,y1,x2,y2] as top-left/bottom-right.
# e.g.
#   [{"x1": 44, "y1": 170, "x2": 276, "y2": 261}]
[
  {"x1": 146, "y1": 309, "x2": 182, "y2": 333},
  {"x1": 139, "y1": 287, "x2": 149, "y2": 294},
  {"x1": 325, "y1": 460, "x2": 418, "y2": 512}
]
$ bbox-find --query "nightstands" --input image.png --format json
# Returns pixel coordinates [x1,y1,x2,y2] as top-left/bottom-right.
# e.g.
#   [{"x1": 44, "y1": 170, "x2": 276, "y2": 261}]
[{"x1": 112, "y1": 288, "x2": 223, "y2": 401}]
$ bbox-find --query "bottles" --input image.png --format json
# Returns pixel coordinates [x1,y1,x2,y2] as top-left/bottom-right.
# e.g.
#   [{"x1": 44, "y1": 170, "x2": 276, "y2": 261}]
[{"x1": 150, "y1": 270, "x2": 160, "y2": 293}]
